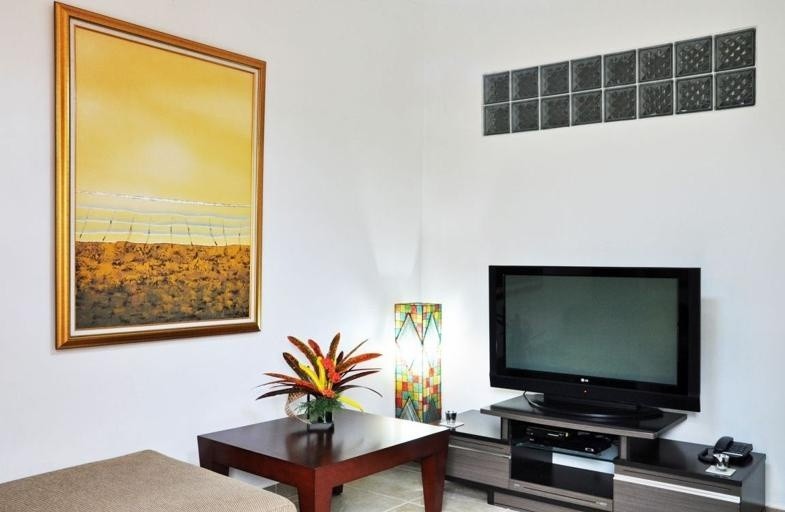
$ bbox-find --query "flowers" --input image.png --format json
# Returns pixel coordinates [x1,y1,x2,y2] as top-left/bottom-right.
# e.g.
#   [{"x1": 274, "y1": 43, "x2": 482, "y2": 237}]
[{"x1": 255, "y1": 331, "x2": 385, "y2": 425}]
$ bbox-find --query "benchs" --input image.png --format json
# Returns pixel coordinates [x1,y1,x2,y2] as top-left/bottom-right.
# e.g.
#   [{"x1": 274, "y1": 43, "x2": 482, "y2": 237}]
[{"x1": 0, "y1": 449, "x2": 298, "y2": 512}]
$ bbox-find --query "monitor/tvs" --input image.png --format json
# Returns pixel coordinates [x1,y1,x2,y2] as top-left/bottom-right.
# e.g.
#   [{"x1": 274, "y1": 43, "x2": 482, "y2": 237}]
[{"x1": 488, "y1": 264, "x2": 702, "y2": 420}]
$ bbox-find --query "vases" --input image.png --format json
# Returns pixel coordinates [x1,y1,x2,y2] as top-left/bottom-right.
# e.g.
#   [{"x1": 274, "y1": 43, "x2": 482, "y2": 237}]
[{"x1": 307, "y1": 390, "x2": 335, "y2": 433}]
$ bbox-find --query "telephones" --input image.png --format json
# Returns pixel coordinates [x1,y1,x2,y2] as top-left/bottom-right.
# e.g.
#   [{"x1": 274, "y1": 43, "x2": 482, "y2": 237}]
[{"x1": 713, "y1": 437, "x2": 753, "y2": 457}]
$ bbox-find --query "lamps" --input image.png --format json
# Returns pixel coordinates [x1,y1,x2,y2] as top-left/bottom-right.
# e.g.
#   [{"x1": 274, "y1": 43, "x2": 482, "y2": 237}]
[{"x1": 395, "y1": 303, "x2": 442, "y2": 464}]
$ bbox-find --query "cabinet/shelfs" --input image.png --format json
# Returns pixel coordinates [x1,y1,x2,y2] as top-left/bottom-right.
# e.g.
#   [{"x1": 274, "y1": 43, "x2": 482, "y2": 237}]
[{"x1": 434, "y1": 409, "x2": 766, "y2": 512}]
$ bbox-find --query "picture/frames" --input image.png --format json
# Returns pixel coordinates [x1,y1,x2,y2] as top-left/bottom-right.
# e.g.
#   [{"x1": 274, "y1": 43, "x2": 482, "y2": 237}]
[{"x1": 53, "y1": 1, "x2": 266, "y2": 350}]
